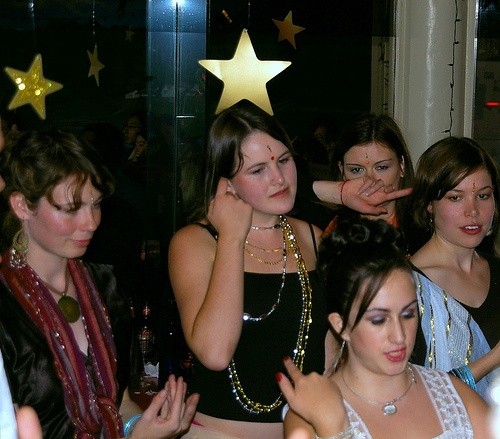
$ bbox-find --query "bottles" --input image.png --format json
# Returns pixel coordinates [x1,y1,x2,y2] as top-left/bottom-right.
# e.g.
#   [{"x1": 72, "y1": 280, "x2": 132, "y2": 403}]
[{"x1": 134, "y1": 300, "x2": 159, "y2": 374}]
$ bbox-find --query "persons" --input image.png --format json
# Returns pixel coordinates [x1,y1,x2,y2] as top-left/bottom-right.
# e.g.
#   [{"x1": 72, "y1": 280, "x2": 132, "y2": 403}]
[{"x1": 0, "y1": 104, "x2": 500, "y2": 439}]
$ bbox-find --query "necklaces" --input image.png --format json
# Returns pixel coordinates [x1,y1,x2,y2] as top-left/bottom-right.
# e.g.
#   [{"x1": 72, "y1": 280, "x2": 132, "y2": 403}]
[
  {"x1": 229, "y1": 209, "x2": 312, "y2": 414},
  {"x1": 23, "y1": 258, "x2": 81, "y2": 322},
  {"x1": 340, "y1": 365, "x2": 415, "y2": 415}
]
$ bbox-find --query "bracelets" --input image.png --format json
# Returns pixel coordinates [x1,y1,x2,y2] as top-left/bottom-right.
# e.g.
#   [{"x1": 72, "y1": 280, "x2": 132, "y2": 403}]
[
  {"x1": 340, "y1": 179, "x2": 350, "y2": 205},
  {"x1": 450, "y1": 364, "x2": 476, "y2": 390},
  {"x1": 123, "y1": 414, "x2": 141, "y2": 437}
]
